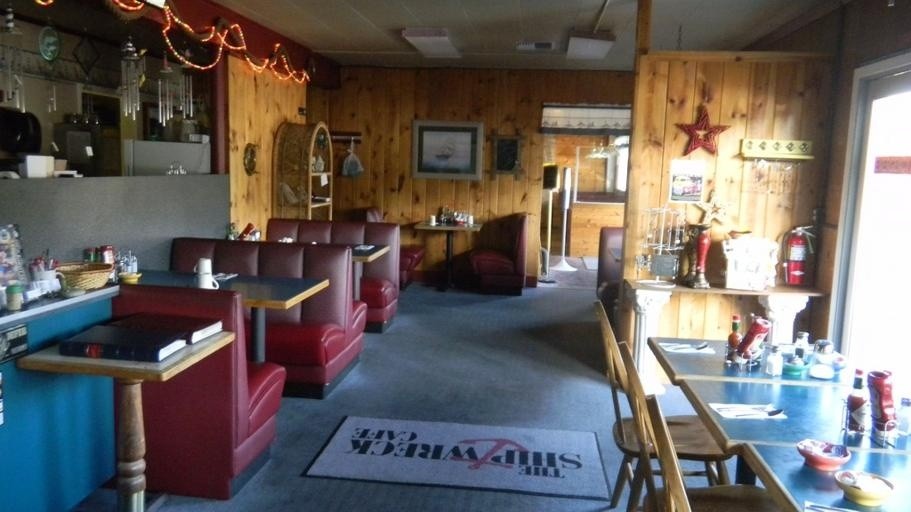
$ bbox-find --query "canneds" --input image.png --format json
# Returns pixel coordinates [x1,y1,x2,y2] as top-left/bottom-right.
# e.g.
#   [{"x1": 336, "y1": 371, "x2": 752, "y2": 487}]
[
  {"x1": 737, "y1": 318, "x2": 772, "y2": 359},
  {"x1": 868, "y1": 372, "x2": 896, "y2": 431}
]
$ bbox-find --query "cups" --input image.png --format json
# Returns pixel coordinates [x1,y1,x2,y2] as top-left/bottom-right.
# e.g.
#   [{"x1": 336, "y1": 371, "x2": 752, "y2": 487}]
[
  {"x1": 194, "y1": 258, "x2": 212, "y2": 275},
  {"x1": 429, "y1": 215, "x2": 436, "y2": 227},
  {"x1": 467, "y1": 216, "x2": 473, "y2": 224},
  {"x1": 198, "y1": 274, "x2": 219, "y2": 290},
  {"x1": 6, "y1": 285, "x2": 23, "y2": 311}
]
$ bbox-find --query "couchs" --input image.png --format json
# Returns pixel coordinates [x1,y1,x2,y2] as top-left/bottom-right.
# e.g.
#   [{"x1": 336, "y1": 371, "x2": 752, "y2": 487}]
[
  {"x1": 113, "y1": 287, "x2": 287, "y2": 500},
  {"x1": 473, "y1": 214, "x2": 528, "y2": 295},
  {"x1": 171, "y1": 236, "x2": 369, "y2": 399},
  {"x1": 355, "y1": 206, "x2": 424, "y2": 287},
  {"x1": 598, "y1": 228, "x2": 625, "y2": 297},
  {"x1": 267, "y1": 218, "x2": 400, "y2": 332}
]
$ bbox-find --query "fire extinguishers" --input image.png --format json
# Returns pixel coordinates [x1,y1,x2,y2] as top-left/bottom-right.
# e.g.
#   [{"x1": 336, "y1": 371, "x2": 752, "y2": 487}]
[{"x1": 782, "y1": 225, "x2": 817, "y2": 285}]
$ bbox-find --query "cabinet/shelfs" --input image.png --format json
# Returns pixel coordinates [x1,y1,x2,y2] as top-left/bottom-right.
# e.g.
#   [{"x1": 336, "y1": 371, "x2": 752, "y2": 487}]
[{"x1": 273, "y1": 121, "x2": 333, "y2": 220}]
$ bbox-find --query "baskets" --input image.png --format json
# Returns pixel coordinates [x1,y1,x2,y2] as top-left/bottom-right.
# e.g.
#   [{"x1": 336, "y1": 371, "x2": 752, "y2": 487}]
[{"x1": 56, "y1": 262, "x2": 114, "y2": 289}]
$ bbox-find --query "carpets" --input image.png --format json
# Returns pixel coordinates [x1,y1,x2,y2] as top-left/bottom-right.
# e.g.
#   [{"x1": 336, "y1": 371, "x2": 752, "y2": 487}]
[{"x1": 301, "y1": 415, "x2": 612, "y2": 501}]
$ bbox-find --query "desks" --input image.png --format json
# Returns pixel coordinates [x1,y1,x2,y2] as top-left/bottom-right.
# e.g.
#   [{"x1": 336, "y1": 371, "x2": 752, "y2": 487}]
[
  {"x1": 0, "y1": 285, "x2": 121, "y2": 512},
  {"x1": 672, "y1": 286, "x2": 825, "y2": 345},
  {"x1": 15, "y1": 329, "x2": 235, "y2": 512}
]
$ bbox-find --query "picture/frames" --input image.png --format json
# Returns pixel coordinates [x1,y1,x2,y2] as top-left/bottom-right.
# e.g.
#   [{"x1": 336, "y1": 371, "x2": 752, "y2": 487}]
[{"x1": 72, "y1": 33, "x2": 101, "y2": 75}]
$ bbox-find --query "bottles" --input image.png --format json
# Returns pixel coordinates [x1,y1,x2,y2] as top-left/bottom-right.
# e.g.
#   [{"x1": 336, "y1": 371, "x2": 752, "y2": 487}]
[
  {"x1": 867, "y1": 367, "x2": 897, "y2": 432},
  {"x1": 894, "y1": 397, "x2": 911, "y2": 453},
  {"x1": 728, "y1": 314, "x2": 834, "y2": 379},
  {"x1": 843, "y1": 368, "x2": 866, "y2": 447}
]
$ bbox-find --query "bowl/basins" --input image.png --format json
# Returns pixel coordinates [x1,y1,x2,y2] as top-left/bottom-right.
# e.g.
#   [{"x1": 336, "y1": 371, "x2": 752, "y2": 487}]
[
  {"x1": 797, "y1": 438, "x2": 850, "y2": 472},
  {"x1": 834, "y1": 471, "x2": 896, "y2": 506}
]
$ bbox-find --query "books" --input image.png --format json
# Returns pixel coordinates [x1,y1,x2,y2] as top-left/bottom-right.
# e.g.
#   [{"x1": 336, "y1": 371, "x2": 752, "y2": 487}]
[
  {"x1": 106, "y1": 312, "x2": 223, "y2": 344},
  {"x1": 59, "y1": 324, "x2": 187, "y2": 363}
]
